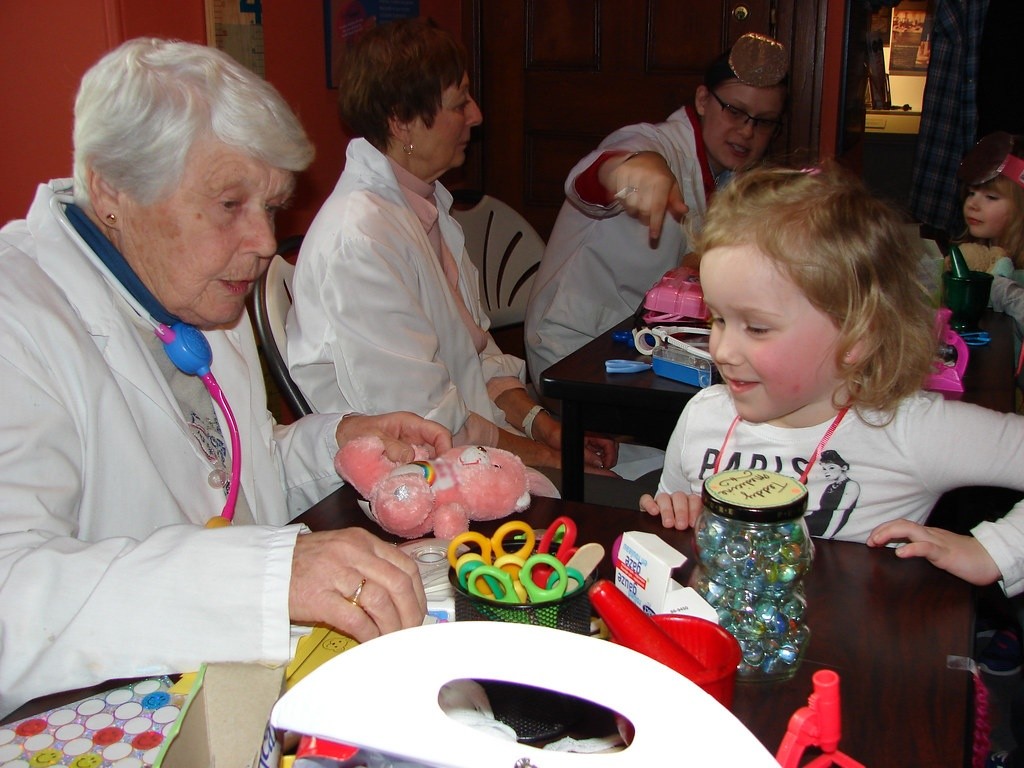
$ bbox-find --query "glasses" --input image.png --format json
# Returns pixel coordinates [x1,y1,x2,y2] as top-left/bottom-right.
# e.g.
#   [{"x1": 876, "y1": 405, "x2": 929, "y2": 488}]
[{"x1": 710, "y1": 93, "x2": 781, "y2": 136}]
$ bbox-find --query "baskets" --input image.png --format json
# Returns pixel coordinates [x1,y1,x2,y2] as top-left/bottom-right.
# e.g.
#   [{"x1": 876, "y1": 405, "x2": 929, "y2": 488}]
[{"x1": 447, "y1": 540, "x2": 593, "y2": 743}]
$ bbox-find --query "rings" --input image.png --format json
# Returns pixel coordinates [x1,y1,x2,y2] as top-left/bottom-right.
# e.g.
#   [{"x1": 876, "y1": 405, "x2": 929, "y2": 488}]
[
  {"x1": 350, "y1": 578, "x2": 367, "y2": 605},
  {"x1": 614, "y1": 184, "x2": 639, "y2": 201}
]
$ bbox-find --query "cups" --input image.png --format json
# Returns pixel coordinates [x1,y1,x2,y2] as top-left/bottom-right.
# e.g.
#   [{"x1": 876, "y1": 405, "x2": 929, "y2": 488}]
[
  {"x1": 610, "y1": 614, "x2": 741, "y2": 713},
  {"x1": 940, "y1": 270, "x2": 995, "y2": 330}
]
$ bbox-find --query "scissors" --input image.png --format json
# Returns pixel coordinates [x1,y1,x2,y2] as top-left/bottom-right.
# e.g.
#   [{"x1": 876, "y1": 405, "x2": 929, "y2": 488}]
[
  {"x1": 605, "y1": 360, "x2": 654, "y2": 373},
  {"x1": 446, "y1": 516, "x2": 585, "y2": 629},
  {"x1": 613, "y1": 331, "x2": 656, "y2": 346},
  {"x1": 959, "y1": 331, "x2": 992, "y2": 346}
]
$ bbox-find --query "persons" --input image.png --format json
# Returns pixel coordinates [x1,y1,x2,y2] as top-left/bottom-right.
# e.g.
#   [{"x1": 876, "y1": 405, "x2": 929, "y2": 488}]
[
  {"x1": 286, "y1": 19, "x2": 620, "y2": 478},
  {"x1": 0, "y1": 38, "x2": 454, "y2": 726},
  {"x1": 524, "y1": 43, "x2": 790, "y2": 448},
  {"x1": 639, "y1": 166, "x2": 1024, "y2": 599},
  {"x1": 943, "y1": 132, "x2": 1024, "y2": 396}
]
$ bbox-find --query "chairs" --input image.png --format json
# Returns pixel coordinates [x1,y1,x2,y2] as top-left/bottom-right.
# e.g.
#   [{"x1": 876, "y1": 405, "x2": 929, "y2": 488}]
[
  {"x1": 254, "y1": 235, "x2": 308, "y2": 420},
  {"x1": 447, "y1": 190, "x2": 546, "y2": 329}
]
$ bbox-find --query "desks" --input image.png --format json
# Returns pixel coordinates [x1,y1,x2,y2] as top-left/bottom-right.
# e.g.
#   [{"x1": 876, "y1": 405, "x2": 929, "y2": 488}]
[
  {"x1": 0, "y1": 467, "x2": 977, "y2": 768},
  {"x1": 538, "y1": 305, "x2": 1013, "y2": 503}
]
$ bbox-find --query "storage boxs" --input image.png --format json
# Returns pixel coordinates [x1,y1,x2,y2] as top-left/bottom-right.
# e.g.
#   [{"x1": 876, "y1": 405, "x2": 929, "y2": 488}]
[
  {"x1": 153, "y1": 659, "x2": 282, "y2": 768},
  {"x1": 865, "y1": 0, "x2": 934, "y2": 135},
  {"x1": 611, "y1": 529, "x2": 720, "y2": 624}
]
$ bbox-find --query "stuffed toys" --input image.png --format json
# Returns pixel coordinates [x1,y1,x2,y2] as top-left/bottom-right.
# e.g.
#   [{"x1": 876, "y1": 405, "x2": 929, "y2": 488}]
[
  {"x1": 333, "y1": 434, "x2": 562, "y2": 541},
  {"x1": 944, "y1": 243, "x2": 1014, "y2": 280}
]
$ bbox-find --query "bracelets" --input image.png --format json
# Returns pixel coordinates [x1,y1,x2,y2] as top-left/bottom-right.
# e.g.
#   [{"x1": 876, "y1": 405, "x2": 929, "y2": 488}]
[{"x1": 522, "y1": 405, "x2": 551, "y2": 441}]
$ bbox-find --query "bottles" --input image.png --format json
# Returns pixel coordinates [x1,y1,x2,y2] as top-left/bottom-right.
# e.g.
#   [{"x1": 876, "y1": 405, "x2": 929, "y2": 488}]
[{"x1": 689, "y1": 470, "x2": 816, "y2": 689}]
